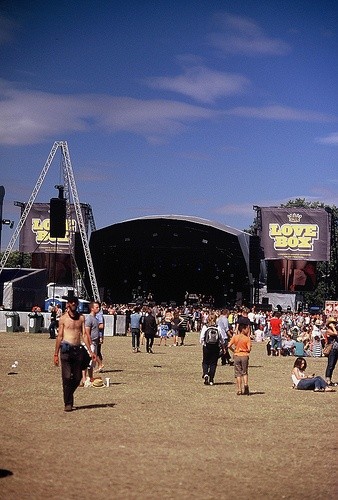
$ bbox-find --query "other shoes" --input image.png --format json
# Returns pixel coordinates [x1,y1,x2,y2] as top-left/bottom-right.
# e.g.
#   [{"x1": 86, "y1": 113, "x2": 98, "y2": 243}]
[
  {"x1": 325, "y1": 388, "x2": 336, "y2": 392},
  {"x1": 237, "y1": 391, "x2": 242, "y2": 395},
  {"x1": 244, "y1": 385, "x2": 249, "y2": 395},
  {"x1": 209, "y1": 382, "x2": 213, "y2": 386},
  {"x1": 314, "y1": 389, "x2": 324, "y2": 392},
  {"x1": 64, "y1": 405, "x2": 71, "y2": 411},
  {"x1": 204, "y1": 375, "x2": 209, "y2": 384},
  {"x1": 326, "y1": 380, "x2": 331, "y2": 385}
]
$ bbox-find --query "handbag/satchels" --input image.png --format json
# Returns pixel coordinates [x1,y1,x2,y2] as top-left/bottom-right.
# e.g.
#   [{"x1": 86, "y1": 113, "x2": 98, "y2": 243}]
[{"x1": 323, "y1": 343, "x2": 333, "y2": 354}]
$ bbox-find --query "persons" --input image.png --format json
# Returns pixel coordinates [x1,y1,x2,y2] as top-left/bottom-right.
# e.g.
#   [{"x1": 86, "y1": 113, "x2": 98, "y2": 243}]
[
  {"x1": 322, "y1": 317, "x2": 338, "y2": 386},
  {"x1": 48, "y1": 302, "x2": 105, "y2": 387},
  {"x1": 53, "y1": 296, "x2": 96, "y2": 411},
  {"x1": 171, "y1": 307, "x2": 187, "y2": 347},
  {"x1": 218, "y1": 309, "x2": 233, "y2": 366},
  {"x1": 292, "y1": 358, "x2": 336, "y2": 392},
  {"x1": 158, "y1": 321, "x2": 169, "y2": 346},
  {"x1": 266, "y1": 326, "x2": 328, "y2": 357},
  {"x1": 142, "y1": 309, "x2": 158, "y2": 353},
  {"x1": 270, "y1": 312, "x2": 284, "y2": 357},
  {"x1": 102, "y1": 303, "x2": 338, "y2": 342},
  {"x1": 200, "y1": 314, "x2": 227, "y2": 387},
  {"x1": 227, "y1": 322, "x2": 252, "y2": 395},
  {"x1": 129, "y1": 307, "x2": 142, "y2": 352}
]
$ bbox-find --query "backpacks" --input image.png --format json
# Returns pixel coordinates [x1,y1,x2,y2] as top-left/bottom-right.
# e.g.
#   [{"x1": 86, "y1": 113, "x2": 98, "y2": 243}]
[{"x1": 204, "y1": 324, "x2": 219, "y2": 345}]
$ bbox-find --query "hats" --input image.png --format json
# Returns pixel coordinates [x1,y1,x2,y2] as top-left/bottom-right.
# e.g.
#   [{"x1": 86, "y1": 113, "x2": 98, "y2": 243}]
[
  {"x1": 92, "y1": 379, "x2": 105, "y2": 388},
  {"x1": 326, "y1": 317, "x2": 337, "y2": 326},
  {"x1": 67, "y1": 297, "x2": 79, "y2": 303}
]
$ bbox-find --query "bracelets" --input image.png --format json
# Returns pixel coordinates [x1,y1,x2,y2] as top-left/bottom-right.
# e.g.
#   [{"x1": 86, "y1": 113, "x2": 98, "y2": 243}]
[
  {"x1": 54, "y1": 355, "x2": 59, "y2": 357},
  {"x1": 307, "y1": 376, "x2": 308, "y2": 379}
]
